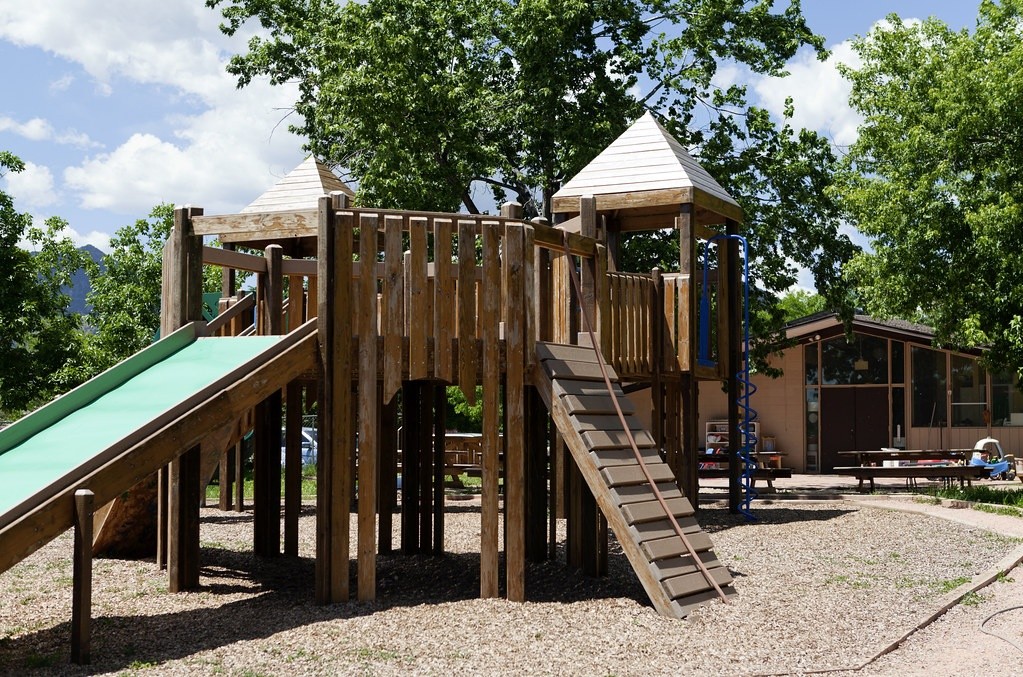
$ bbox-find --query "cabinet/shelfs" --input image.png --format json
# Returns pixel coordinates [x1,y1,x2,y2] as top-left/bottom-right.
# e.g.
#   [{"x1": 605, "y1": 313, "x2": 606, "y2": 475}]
[{"x1": 705, "y1": 422, "x2": 761, "y2": 452}]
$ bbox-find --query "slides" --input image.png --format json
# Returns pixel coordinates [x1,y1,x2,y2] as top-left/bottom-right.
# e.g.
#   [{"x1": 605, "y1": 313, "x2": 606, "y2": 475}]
[{"x1": 1, "y1": 319, "x2": 319, "y2": 576}]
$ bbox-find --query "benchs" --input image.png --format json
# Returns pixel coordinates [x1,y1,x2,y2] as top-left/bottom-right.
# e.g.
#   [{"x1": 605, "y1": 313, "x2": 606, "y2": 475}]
[
  {"x1": 699, "y1": 466, "x2": 797, "y2": 479},
  {"x1": 831, "y1": 464, "x2": 995, "y2": 479}
]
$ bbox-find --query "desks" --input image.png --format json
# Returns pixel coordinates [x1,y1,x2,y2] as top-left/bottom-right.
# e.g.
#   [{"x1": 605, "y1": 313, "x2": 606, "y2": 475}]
[
  {"x1": 837, "y1": 449, "x2": 988, "y2": 490},
  {"x1": 698, "y1": 450, "x2": 789, "y2": 493}
]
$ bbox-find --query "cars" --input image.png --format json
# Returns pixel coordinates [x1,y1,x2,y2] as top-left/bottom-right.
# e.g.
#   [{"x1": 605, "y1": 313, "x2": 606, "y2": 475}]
[{"x1": 280, "y1": 427, "x2": 318, "y2": 466}]
